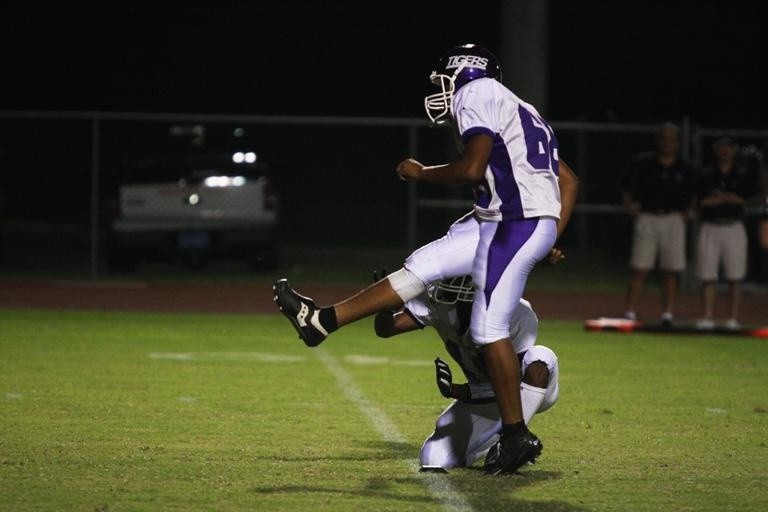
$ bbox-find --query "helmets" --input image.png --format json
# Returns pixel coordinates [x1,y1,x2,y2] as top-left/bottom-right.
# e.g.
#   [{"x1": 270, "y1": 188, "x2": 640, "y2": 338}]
[
  {"x1": 424, "y1": 42, "x2": 503, "y2": 124},
  {"x1": 427, "y1": 276, "x2": 474, "y2": 304}
]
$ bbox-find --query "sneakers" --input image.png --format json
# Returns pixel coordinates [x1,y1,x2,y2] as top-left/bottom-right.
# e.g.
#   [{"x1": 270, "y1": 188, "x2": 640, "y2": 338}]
[
  {"x1": 481, "y1": 431, "x2": 543, "y2": 476},
  {"x1": 273, "y1": 279, "x2": 329, "y2": 347}
]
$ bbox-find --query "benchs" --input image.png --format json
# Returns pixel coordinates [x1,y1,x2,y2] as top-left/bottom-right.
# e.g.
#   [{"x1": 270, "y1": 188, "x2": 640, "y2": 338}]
[{"x1": 110, "y1": 179, "x2": 272, "y2": 261}]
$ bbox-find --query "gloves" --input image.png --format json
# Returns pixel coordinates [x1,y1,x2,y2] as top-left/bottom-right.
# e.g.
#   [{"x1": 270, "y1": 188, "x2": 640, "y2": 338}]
[{"x1": 434, "y1": 357, "x2": 452, "y2": 397}]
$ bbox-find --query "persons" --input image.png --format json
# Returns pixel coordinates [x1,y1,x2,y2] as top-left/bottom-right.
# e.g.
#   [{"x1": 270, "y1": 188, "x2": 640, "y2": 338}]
[
  {"x1": 268, "y1": 40, "x2": 582, "y2": 477},
  {"x1": 372, "y1": 269, "x2": 559, "y2": 473},
  {"x1": 622, "y1": 120, "x2": 700, "y2": 323},
  {"x1": 695, "y1": 132, "x2": 747, "y2": 330},
  {"x1": 757, "y1": 197, "x2": 767, "y2": 250}
]
